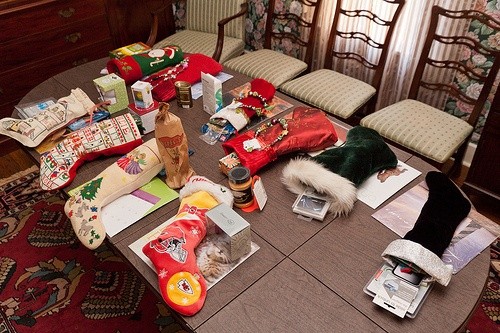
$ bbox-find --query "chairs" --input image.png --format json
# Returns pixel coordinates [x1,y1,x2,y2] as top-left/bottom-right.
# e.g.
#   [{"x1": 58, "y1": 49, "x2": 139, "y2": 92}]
[
  {"x1": 223, "y1": 0, "x2": 322, "y2": 91},
  {"x1": 145, "y1": 0, "x2": 249, "y2": 65},
  {"x1": 360, "y1": 5, "x2": 500, "y2": 178},
  {"x1": 280, "y1": 0, "x2": 405, "y2": 123}
]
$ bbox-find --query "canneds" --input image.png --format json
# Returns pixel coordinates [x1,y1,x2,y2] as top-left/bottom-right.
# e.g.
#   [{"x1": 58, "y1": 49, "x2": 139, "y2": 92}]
[
  {"x1": 228, "y1": 167, "x2": 254, "y2": 208},
  {"x1": 175, "y1": 81, "x2": 193, "y2": 109}
]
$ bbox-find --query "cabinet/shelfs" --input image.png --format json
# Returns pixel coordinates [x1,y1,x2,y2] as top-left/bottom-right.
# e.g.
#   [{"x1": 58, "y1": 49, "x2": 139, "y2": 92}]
[{"x1": 0, "y1": 0, "x2": 179, "y2": 157}]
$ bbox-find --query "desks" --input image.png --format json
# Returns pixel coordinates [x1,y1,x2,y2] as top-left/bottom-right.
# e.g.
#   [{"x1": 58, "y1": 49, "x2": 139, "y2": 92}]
[{"x1": 11, "y1": 52, "x2": 490, "y2": 333}]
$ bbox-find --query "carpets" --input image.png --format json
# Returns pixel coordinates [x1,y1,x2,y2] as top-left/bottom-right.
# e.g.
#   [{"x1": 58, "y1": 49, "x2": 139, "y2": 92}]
[{"x1": 0, "y1": 164, "x2": 500, "y2": 333}]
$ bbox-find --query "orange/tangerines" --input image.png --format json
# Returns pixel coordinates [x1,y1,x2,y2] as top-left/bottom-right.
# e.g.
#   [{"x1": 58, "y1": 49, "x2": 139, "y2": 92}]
[{"x1": 167, "y1": 272, "x2": 202, "y2": 307}]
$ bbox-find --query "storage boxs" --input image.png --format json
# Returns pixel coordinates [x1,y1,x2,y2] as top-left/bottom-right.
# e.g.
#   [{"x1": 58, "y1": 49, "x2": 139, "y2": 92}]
[
  {"x1": 93, "y1": 73, "x2": 160, "y2": 134},
  {"x1": 204, "y1": 202, "x2": 251, "y2": 260},
  {"x1": 201, "y1": 71, "x2": 222, "y2": 116}
]
